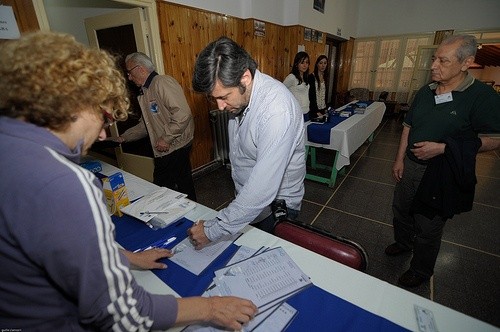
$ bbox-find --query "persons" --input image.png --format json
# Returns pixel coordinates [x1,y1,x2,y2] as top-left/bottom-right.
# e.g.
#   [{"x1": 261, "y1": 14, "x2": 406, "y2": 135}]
[
  {"x1": 282, "y1": 51, "x2": 310, "y2": 122},
  {"x1": 0, "y1": 31, "x2": 258, "y2": 332},
  {"x1": 384, "y1": 35, "x2": 500, "y2": 290},
  {"x1": 186, "y1": 35, "x2": 306, "y2": 250},
  {"x1": 308, "y1": 55, "x2": 329, "y2": 119},
  {"x1": 114, "y1": 52, "x2": 197, "y2": 202}
]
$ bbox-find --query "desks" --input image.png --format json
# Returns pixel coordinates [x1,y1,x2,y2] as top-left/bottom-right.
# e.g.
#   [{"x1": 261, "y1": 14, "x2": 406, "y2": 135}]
[
  {"x1": 304, "y1": 100, "x2": 386, "y2": 189},
  {"x1": 81, "y1": 159, "x2": 500, "y2": 332}
]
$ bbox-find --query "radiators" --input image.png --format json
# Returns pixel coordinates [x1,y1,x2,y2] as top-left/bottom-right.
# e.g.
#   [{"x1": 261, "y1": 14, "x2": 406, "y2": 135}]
[{"x1": 210, "y1": 109, "x2": 231, "y2": 173}]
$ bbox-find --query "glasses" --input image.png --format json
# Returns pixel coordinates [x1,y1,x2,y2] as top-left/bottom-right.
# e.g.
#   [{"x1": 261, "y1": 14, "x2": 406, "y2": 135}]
[
  {"x1": 127, "y1": 65, "x2": 138, "y2": 76},
  {"x1": 98, "y1": 104, "x2": 117, "y2": 127}
]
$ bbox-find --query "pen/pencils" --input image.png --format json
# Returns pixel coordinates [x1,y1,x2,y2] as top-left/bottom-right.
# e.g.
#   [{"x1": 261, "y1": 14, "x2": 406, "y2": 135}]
[
  {"x1": 140, "y1": 212, "x2": 168, "y2": 214},
  {"x1": 195, "y1": 219, "x2": 200, "y2": 227},
  {"x1": 133, "y1": 235, "x2": 178, "y2": 253}
]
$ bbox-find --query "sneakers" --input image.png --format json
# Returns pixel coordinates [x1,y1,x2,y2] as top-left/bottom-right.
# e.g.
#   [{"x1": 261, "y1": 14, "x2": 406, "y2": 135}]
[
  {"x1": 399, "y1": 269, "x2": 430, "y2": 287},
  {"x1": 385, "y1": 243, "x2": 408, "y2": 256}
]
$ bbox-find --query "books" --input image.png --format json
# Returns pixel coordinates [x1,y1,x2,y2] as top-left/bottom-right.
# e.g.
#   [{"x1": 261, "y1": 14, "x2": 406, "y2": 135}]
[{"x1": 150, "y1": 199, "x2": 197, "y2": 228}]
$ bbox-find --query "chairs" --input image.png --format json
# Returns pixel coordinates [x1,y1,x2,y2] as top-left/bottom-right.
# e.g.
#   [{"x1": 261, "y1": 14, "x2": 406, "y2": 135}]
[
  {"x1": 274, "y1": 219, "x2": 369, "y2": 273},
  {"x1": 346, "y1": 88, "x2": 370, "y2": 101},
  {"x1": 395, "y1": 95, "x2": 415, "y2": 126}
]
